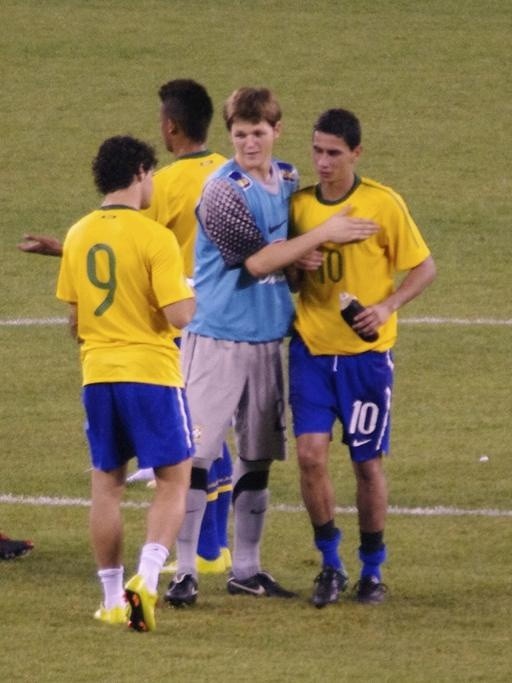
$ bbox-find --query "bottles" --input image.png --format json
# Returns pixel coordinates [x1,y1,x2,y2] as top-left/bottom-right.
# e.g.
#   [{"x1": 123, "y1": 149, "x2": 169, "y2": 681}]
[{"x1": 339, "y1": 292, "x2": 379, "y2": 343}]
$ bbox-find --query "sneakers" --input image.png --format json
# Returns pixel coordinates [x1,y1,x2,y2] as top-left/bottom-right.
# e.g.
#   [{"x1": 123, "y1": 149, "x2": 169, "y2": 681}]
[
  {"x1": 92, "y1": 603, "x2": 127, "y2": 625},
  {"x1": 225, "y1": 571, "x2": 300, "y2": 599},
  {"x1": 124, "y1": 575, "x2": 157, "y2": 633},
  {"x1": 165, "y1": 570, "x2": 198, "y2": 605},
  {"x1": 1, "y1": 534, "x2": 35, "y2": 559},
  {"x1": 355, "y1": 576, "x2": 385, "y2": 604},
  {"x1": 160, "y1": 547, "x2": 231, "y2": 573},
  {"x1": 311, "y1": 565, "x2": 349, "y2": 608}
]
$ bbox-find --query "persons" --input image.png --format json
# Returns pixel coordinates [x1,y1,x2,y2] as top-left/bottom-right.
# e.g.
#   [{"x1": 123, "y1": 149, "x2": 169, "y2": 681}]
[
  {"x1": 163, "y1": 86, "x2": 379, "y2": 608},
  {"x1": 16, "y1": 79, "x2": 234, "y2": 576},
  {"x1": 55, "y1": 134, "x2": 195, "y2": 634},
  {"x1": 287, "y1": 109, "x2": 438, "y2": 608}
]
